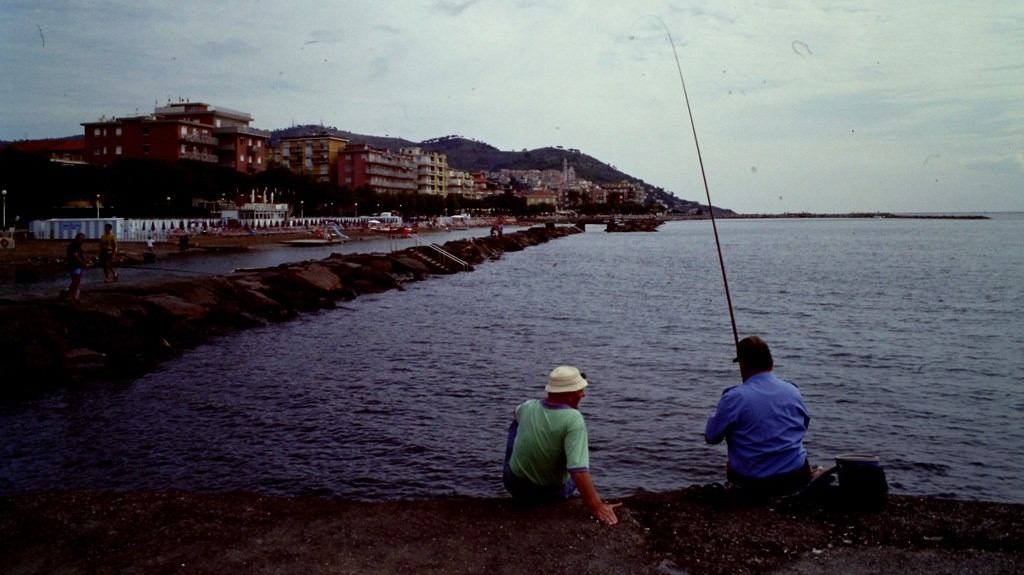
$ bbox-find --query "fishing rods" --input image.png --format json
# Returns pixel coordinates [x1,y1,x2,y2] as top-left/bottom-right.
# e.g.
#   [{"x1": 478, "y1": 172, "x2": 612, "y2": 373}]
[{"x1": 655, "y1": 14, "x2": 739, "y2": 353}]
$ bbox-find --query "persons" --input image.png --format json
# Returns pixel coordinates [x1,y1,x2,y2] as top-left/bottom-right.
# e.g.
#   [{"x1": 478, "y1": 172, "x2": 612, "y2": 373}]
[
  {"x1": 147, "y1": 236, "x2": 154, "y2": 250},
  {"x1": 490, "y1": 222, "x2": 504, "y2": 237},
  {"x1": 67, "y1": 233, "x2": 94, "y2": 303},
  {"x1": 502, "y1": 366, "x2": 622, "y2": 528},
  {"x1": 705, "y1": 335, "x2": 811, "y2": 485},
  {"x1": 99, "y1": 224, "x2": 119, "y2": 281}
]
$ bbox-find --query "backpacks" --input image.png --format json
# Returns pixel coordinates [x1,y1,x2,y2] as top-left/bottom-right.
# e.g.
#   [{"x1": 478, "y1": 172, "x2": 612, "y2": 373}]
[{"x1": 812, "y1": 462, "x2": 889, "y2": 514}]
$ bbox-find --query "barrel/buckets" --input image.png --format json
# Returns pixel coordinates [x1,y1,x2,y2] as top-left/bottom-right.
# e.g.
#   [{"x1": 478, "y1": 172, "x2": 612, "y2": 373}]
[{"x1": 834, "y1": 453, "x2": 882, "y2": 511}]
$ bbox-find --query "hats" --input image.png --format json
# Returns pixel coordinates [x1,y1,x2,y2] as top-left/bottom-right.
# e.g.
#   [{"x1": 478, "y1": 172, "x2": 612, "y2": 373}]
[
  {"x1": 733, "y1": 337, "x2": 768, "y2": 363},
  {"x1": 545, "y1": 365, "x2": 588, "y2": 392}
]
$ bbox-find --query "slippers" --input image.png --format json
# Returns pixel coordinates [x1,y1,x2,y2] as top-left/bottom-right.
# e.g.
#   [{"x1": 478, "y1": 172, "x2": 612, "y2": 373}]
[{"x1": 682, "y1": 483, "x2": 733, "y2": 504}]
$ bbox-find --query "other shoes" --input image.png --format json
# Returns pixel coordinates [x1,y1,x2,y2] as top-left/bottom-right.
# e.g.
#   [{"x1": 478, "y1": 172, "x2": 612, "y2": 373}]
[
  {"x1": 66, "y1": 297, "x2": 83, "y2": 304},
  {"x1": 104, "y1": 279, "x2": 110, "y2": 282},
  {"x1": 113, "y1": 273, "x2": 118, "y2": 281}
]
